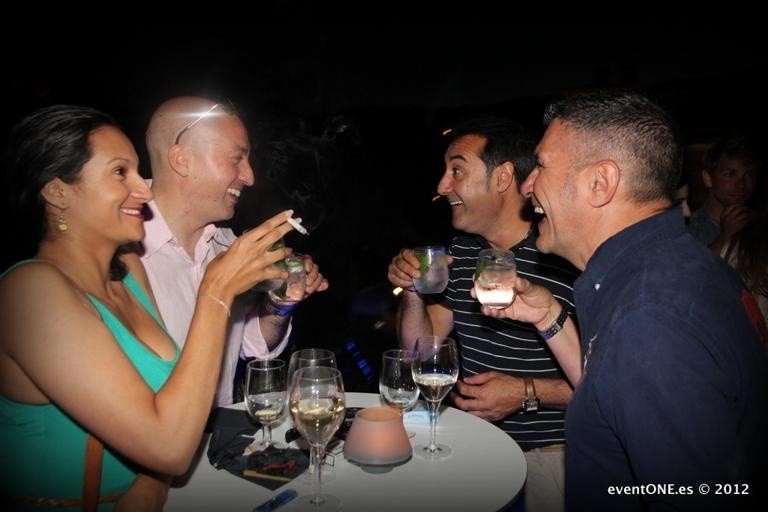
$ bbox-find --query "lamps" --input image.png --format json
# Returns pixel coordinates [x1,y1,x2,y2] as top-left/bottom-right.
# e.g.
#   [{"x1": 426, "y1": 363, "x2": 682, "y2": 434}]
[{"x1": 344, "y1": 408, "x2": 412, "y2": 473}]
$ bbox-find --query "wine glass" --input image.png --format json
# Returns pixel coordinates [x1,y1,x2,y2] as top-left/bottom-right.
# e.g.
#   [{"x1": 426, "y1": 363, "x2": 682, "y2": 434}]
[
  {"x1": 244, "y1": 358, "x2": 289, "y2": 453},
  {"x1": 283, "y1": 366, "x2": 348, "y2": 511},
  {"x1": 379, "y1": 348, "x2": 422, "y2": 441},
  {"x1": 284, "y1": 347, "x2": 341, "y2": 483},
  {"x1": 406, "y1": 336, "x2": 462, "y2": 462}
]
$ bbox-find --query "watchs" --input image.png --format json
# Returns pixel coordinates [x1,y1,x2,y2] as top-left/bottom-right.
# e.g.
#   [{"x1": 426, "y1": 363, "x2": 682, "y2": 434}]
[{"x1": 522, "y1": 375, "x2": 540, "y2": 416}]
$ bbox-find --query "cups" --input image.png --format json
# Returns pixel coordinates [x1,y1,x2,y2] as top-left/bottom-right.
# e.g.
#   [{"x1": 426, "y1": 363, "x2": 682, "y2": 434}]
[
  {"x1": 241, "y1": 228, "x2": 286, "y2": 292},
  {"x1": 410, "y1": 246, "x2": 451, "y2": 296},
  {"x1": 265, "y1": 251, "x2": 306, "y2": 305},
  {"x1": 473, "y1": 248, "x2": 519, "y2": 310}
]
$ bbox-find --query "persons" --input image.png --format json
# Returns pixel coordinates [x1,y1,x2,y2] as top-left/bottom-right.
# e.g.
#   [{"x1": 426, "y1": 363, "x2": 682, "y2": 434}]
[
  {"x1": 129, "y1": 95, "x2": 329, "y2": 409},
  {"x1": 386, "y1": 113, "x2": 582, "y2": 512},
  {"x1": 669, "y1": 134, "x2": 768, "y2": 322},
  {"x1": 0, "y1": 107, "x2": 302, "y2": 512},
  {"x1": 471, "y1": 72, "x2": 767, "y2": 512}
]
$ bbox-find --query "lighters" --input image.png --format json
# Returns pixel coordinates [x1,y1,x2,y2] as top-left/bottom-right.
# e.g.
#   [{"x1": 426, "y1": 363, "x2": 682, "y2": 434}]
[{"x1": 252, "y1": 487, "x2": 297, "y2": 512}]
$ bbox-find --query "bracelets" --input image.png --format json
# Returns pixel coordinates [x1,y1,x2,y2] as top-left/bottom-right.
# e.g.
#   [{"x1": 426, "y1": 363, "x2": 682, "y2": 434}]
[
  {"x1": 537, "y1": 306, "x2": 568, "y2": 340},
  {"x1": 264, "y1": 302, "x2": 295, "y2": 317},
  {"x1": 199, "y1": 292, "x2": 233, "y2": 319}
]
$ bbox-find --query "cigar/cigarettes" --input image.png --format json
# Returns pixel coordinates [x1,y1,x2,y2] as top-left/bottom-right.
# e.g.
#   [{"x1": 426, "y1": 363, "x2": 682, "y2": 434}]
[{"x1": 286, "y1": 215, "x2": 309, "y2": 236}]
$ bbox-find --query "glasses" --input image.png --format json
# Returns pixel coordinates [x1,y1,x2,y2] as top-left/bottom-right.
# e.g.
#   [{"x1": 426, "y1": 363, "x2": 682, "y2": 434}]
[{"x1": 172, "y1": 93, "x2": 242, "y2": 148}]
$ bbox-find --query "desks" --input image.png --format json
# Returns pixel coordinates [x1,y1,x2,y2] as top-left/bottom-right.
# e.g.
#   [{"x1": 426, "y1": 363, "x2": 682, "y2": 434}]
[{"x1": 163, "y1": 390, "x2": 528, "y2": 510}]
